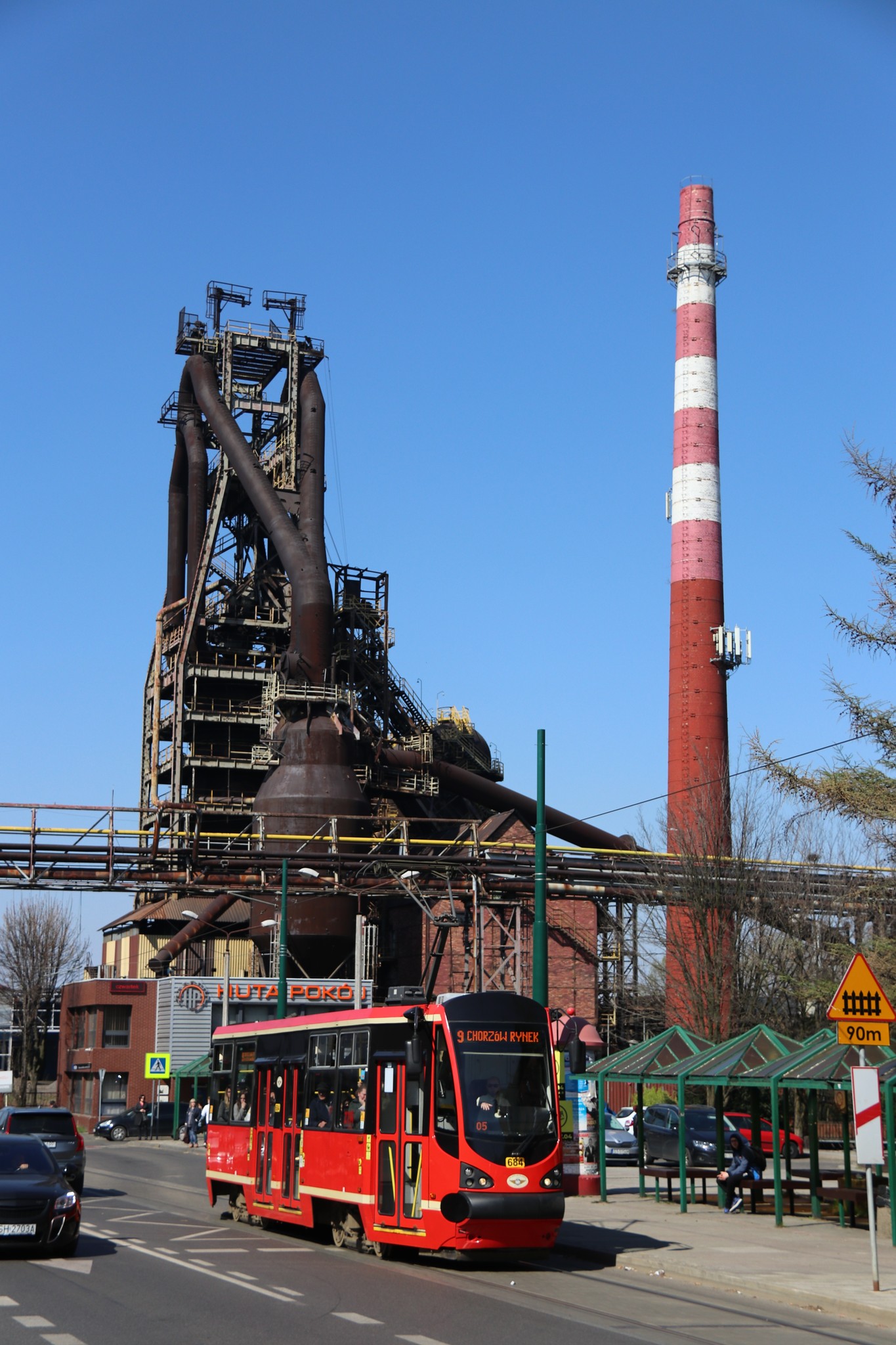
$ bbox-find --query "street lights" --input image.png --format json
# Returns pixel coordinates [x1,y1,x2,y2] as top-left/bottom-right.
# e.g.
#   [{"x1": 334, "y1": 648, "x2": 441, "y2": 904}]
[
  {"x1": 181, "y1": 910, "x2": 280, "y2": 1031},
  {"x1": 298, "y1": 867, "x2": 423, "y2": 1013}
]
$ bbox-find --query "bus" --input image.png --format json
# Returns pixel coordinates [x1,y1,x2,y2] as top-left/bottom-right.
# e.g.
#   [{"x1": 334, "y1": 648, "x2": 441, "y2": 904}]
[{"x1": 202, "y1": 859, "x2": 588, "y2": 1265}]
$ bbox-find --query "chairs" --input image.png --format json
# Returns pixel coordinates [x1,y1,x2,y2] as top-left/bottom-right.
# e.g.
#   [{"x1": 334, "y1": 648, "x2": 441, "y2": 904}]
[{"x1": 467, "y1": 1080, "x2": 491, "y2": 1123}]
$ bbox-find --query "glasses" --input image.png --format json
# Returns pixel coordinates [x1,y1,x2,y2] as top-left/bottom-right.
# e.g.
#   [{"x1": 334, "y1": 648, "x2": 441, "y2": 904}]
[{"x1": 730, "y1": 1140, "x2": 737, "y2": 1143}]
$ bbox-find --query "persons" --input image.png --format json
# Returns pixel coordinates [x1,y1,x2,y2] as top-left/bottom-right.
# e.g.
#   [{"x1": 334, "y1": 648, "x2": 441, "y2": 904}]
[
  {"x1": 475, "y1": 1075, "x2": 511, "y2": 1123},
  {"x1": 579, "y1": 1138, "x2": 598, "y2": 1163},
  {"x1": 521, "y1": 1067, "x2": 546, "y2": 1108},
  {"x1": 49, "y1": 1100, "x2": 58, "y2": 1108},
  {"x1": 215, "y1": 1079, "x2": 367, "y2": 1132},
  {"x1": 185, "y1": 1097, "x2": 210, "y2": 1147},
  {"x1": 135, "y1": 1094, "x2": 150, "y2": 1140},
  {"x1": 716, "y1": 1134, "x2": 767, "y2": 1213}
]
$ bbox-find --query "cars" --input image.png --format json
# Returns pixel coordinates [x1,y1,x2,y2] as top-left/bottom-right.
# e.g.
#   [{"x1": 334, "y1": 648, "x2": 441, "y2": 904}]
[
  {"x1": 642, "y1": 1103, "x2": 751, "y2": 1168},
  {"x1": 723, "y1": 1112, "x2": 804, "y2": 1158},
  {"x1": 93, "y1": 1101, "x2": 203, "y2": 1143},
  {"x1": 0, "y1": 1133, "x2": 85, "y2": 1259},
  {"x1": 604, "y1": 1112, "x2": 639, "y2": 1167},
  {"x1": 614, "y1": 1106, "x2": 649, "y2": 1135},
  {"x1": 0, "y1": 1105, "x2": 85, "y2": 1196}
]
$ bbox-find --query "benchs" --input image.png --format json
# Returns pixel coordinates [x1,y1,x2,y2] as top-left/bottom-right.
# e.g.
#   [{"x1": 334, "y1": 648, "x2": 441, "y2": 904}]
[
  {"x1": 640, "y1": 1167, "x2": 720, "y2": 1204},
  {"x1": 734, "y1": 1169, "x2": 874, "y2": 1228}
]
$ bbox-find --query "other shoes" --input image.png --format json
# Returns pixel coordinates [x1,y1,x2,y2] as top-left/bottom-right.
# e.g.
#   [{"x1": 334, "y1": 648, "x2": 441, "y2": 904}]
[
  {"x1": 729, "y1": 1195, "x2": 742, "y2": 1212},
  {"x1": 190, "y1": 1144, "x2": 193, "y2": 1147},
  {"x1": 724, "y1": 1201, "x2": 731, "y2": 1213},
  {"x1": 203, "y1": 1142, "x2": 207, "y2": 1148},
  {"x1": 195, "y1": 1143, "x2": 198, "y2": 1147}
]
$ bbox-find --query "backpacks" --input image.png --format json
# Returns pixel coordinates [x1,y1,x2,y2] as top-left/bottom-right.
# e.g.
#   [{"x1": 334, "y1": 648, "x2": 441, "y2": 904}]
[{"x1": 742, "y1": 1145, "x2": 767, "y2": 1172}]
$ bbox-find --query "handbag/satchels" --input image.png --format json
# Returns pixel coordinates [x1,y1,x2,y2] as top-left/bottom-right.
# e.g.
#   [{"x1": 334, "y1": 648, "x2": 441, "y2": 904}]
[{"x1": 184, "y1": 1126, "x2": 190, "y2": 1143}]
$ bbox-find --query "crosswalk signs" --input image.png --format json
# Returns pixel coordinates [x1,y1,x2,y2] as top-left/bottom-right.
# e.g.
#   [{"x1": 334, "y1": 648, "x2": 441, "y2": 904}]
[{"x1": 145, "y1": 1052, "x2": 171, "y2": 1081}]
